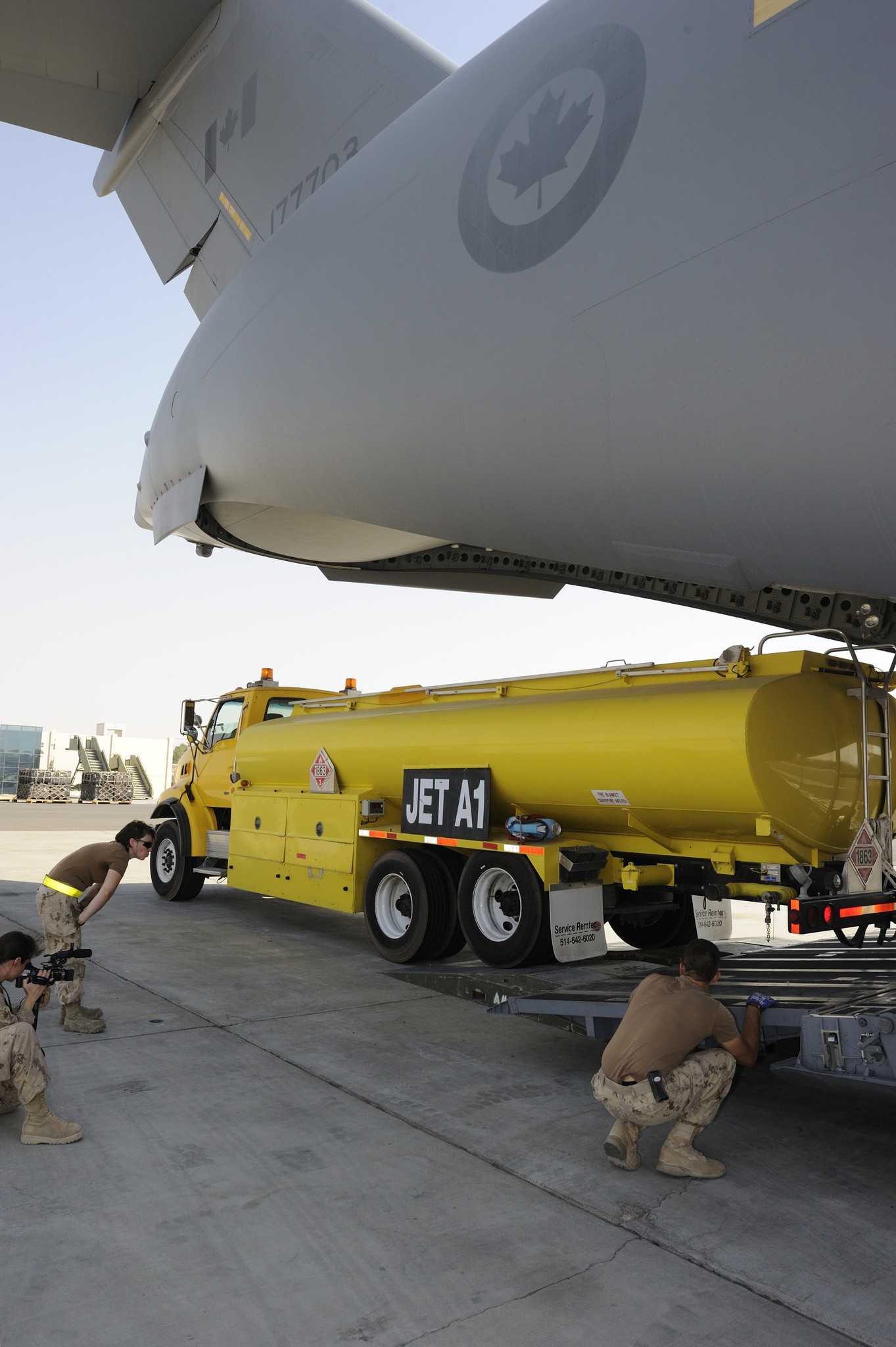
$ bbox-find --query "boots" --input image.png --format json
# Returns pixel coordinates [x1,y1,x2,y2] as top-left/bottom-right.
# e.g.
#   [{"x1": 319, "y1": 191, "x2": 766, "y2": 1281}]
[
  {"x1": 604, "y1": 1119, "x2": 641, "y2": 1171},
  {"x1": 64, "y1": 1000, "x2": 107, "y2": 1034},
  {"x1": 655, "y1": 1120, "x2": 726, "y2": 1178},
  {"x1": 21, "y1": 1091, "x2": 84, "y2": 1145},
  {"x1": 60, "y1": 999, "x2": 104, "y2": 1024}
]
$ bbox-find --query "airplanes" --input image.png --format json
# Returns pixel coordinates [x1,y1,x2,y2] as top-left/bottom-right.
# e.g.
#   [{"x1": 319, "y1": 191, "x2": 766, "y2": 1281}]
[{"x1": 0, "y1": 2, "x2": 896, "y2": 659}]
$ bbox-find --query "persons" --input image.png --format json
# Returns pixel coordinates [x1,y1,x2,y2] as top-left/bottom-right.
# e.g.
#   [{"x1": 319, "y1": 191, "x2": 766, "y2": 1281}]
[
  {"x1": 591, "y1": 939, "x2": 779, "y2": 1179},
  {"x1": 35, "y1": 819, "x2": 158, "y2": 1033},
  {"x1": 0, "y1": 930, "x2": 83, "y2": 1143}
]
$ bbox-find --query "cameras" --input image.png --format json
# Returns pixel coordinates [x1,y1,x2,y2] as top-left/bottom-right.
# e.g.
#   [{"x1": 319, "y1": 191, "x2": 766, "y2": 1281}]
[{"x1": 15, "y1": 962, "x2": 93, "y2": 988}]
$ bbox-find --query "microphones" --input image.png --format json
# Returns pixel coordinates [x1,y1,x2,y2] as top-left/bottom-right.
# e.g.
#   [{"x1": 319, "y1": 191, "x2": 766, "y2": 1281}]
[{"x1": 52, "y1": 949, "x2": 92, "y2": 959}]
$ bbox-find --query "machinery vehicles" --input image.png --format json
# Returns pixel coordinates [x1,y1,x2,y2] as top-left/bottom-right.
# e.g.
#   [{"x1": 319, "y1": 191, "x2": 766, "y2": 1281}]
[{"x1": 146, "y1": 627, "x2": 895, "y2": 966}]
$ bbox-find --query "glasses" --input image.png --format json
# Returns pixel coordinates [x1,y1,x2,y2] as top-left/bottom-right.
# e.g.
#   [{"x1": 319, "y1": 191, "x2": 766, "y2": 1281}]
[{"x1": 135, "y1": 838, "x2": 152, "y2": 848}]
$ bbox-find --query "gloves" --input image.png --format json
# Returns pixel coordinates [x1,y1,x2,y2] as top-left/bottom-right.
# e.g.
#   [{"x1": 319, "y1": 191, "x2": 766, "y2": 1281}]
[
  {"x1": 746, "y1": 992, "x2": 775, "y2": 1013},
  {"x1": 70, "y1": 905, "x2": 85, "y2": 927}
]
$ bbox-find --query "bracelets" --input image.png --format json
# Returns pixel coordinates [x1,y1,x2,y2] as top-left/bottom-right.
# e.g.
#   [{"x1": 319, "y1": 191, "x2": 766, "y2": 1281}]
[{"x1": 76, "y1": 921, "x2": 86, "y2": 926}]
